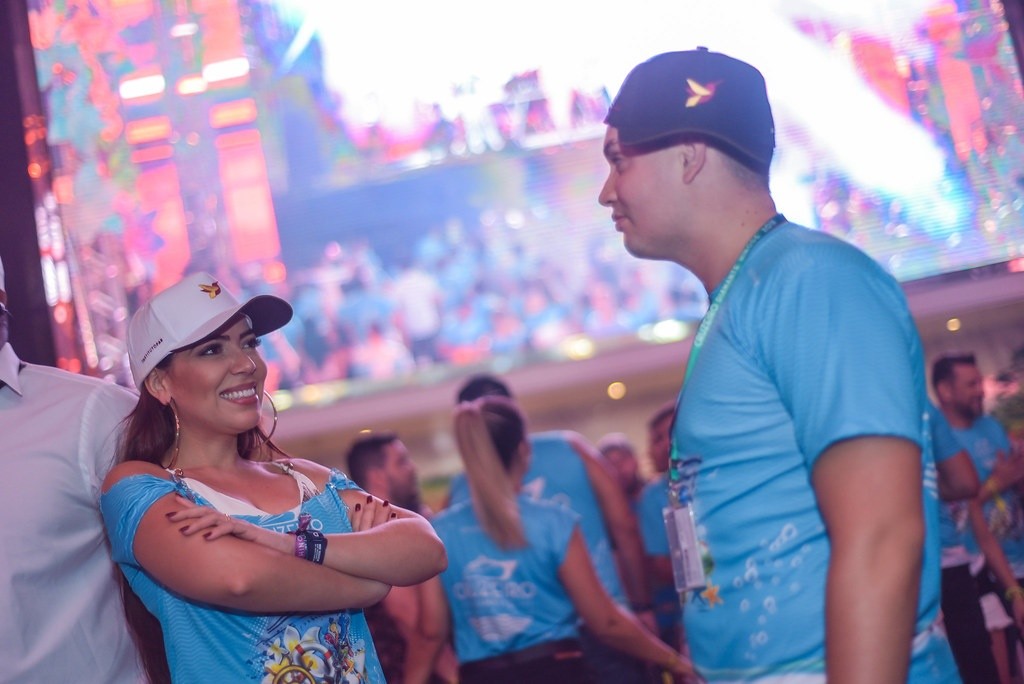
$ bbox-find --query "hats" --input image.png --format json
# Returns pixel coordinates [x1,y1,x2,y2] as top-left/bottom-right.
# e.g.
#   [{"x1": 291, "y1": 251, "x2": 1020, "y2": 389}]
[
  {"x1": 128, "y1": 271, "x2": 293, "y2": 393},
  {"x1": 603, "y1": 45, "x2": 776, "y2": 165}
]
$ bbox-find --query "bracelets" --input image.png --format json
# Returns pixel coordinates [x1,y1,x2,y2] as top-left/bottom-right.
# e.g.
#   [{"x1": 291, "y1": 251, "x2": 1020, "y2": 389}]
[
  {"x1": 987, "y1": 477, "x2": 999, "y2": 497},
  {"x1": 1005, "y1": 587, "x2": 1024, "y2": 601},
  {"x1": 286, "y1": 513, "x2": 327, "y2": 565}
]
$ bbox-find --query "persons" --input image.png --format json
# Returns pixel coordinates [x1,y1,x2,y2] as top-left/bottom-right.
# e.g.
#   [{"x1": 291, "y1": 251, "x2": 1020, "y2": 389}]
[
  {"x1": 0, "y1": 259, "x2": 145, "y2": 684},
  {"x1": 600, "y1": 46, "x2": 968, "y2": 683},
  {"x1": 232, "y1": 233, "x2": 710, "y2": 391},
  {"x1": 935, "y1": 353, "x2": 1024, "y2": 684},
  {"x1": 99, "y1": 271, "x2": 445, "y2": 684},
  {"x1": 145, "y1": 374, "x2": 711, "y2": 683}
]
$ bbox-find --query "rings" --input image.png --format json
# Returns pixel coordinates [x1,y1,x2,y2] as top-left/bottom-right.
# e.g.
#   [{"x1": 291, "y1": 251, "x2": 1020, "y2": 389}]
[{"x1": 225, "y1": 514, "x2": 231, "y2": 522}]
